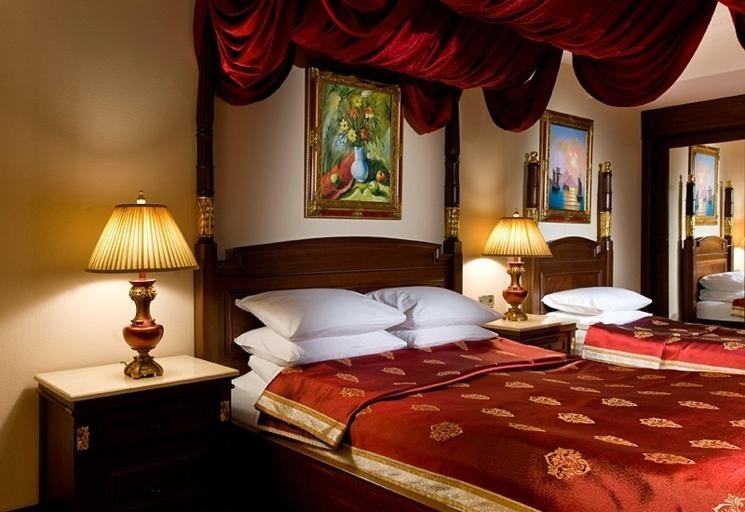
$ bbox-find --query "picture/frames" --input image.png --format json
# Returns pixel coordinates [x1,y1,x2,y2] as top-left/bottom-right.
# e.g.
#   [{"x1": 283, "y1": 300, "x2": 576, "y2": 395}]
[
  {"x1": 303, "y1": 66, "x2": 403, "y2": 222},
  {"x1": 687, "y1": 144, "x2": 720, "y2": 226},
  {"x1": 541, "y1": 108, "x2": 595, "y2": 224}
]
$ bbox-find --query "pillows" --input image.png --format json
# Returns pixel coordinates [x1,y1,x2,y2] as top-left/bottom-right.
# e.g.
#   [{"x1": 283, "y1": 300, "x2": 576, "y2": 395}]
[
  {"x1": 229, "y1": 287, "x2": 407, "y2": 341},
  {"x1": 699, "y1": 271, "x2": 745, "y2": 293},
  {"x1": 364, "y1": 284, "x2": 507, "y2": 329},
  {"x1": 231, "y1": 324, "x2": 411, "y2": 365},
  {"x1": 545, "y1": 309, "x2": 654, "y2": 328},
  {"x1": 387, "y1": 323, "x2": 502, "y2": 351},
  {"x1": 539, "y1": 285, "x2": 653, "y2": 315}
]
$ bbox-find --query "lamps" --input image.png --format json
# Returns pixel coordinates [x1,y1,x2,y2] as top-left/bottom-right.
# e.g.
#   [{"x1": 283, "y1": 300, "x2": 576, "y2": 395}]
[
  {"x1": 85, "y1": 190, "x2": 201, "y2": 381},
  {"x1": 482, "y1": 209, "x2": 554, "y2": 323}
]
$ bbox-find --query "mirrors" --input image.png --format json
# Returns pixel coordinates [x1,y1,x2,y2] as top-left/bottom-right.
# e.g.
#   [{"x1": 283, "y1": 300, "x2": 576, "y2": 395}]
[{"x1": 640, "y1": 91, "x2": 744, "y2": 326}]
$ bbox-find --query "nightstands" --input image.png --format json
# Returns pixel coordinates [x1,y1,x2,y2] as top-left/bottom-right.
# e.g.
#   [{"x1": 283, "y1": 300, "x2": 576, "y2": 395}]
[
  {"x1": 481, "y1": 313, "x2": 578, "y2": 355},
  {"x1": 31, "y1": 354, "x2": 241, "y2": 511}
]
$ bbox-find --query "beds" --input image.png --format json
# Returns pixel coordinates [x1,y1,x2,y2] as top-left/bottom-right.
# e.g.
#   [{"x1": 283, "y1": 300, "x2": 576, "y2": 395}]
[
  {"x1": 521, "y1": 151, "x2": 745, "y2": 377},
  {"x1": 681, "y1": 176, "x2": 744, "y2": 322},
  {"x1": 194, "y1": 235, "x2": 745, "y2": 512}
]
